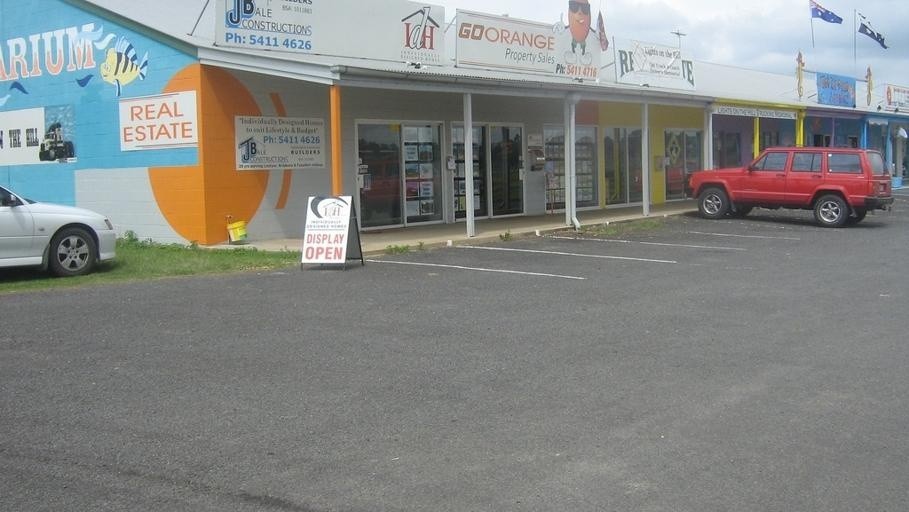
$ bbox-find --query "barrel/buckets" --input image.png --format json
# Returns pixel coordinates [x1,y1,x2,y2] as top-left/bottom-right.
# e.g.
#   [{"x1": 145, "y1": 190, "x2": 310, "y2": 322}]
[{"x1": 226, "y1": 215, "x2": 248, "y2": 243}]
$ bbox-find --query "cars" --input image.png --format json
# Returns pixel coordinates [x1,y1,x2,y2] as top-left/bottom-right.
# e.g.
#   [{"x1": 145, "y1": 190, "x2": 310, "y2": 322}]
[
  {"x1": 366, "y1": 159, "x2": 418, "y2": 217},
  {"x1": 633, "y1": 160, "x2": 698, "y2": 194},
  {"x1": 0, "y1": 185, "x2": 117, "y2": 276},
  {"x1": 686, "y1": 146, "x2": 894, "y2": 227}
]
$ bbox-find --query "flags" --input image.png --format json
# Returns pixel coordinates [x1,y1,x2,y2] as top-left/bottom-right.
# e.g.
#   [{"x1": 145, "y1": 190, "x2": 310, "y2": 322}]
[
  {"x1": 858, "y1": 11, "x2": 890, "y2": 50},
  {"x1": 810, "y1": 0, "x2": 843, "y2": 24}
]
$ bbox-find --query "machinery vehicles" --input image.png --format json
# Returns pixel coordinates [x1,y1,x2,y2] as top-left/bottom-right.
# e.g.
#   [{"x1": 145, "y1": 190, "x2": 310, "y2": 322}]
[{"x1": 39, "y1": 126, "x2": 74, "y2": 160}]
